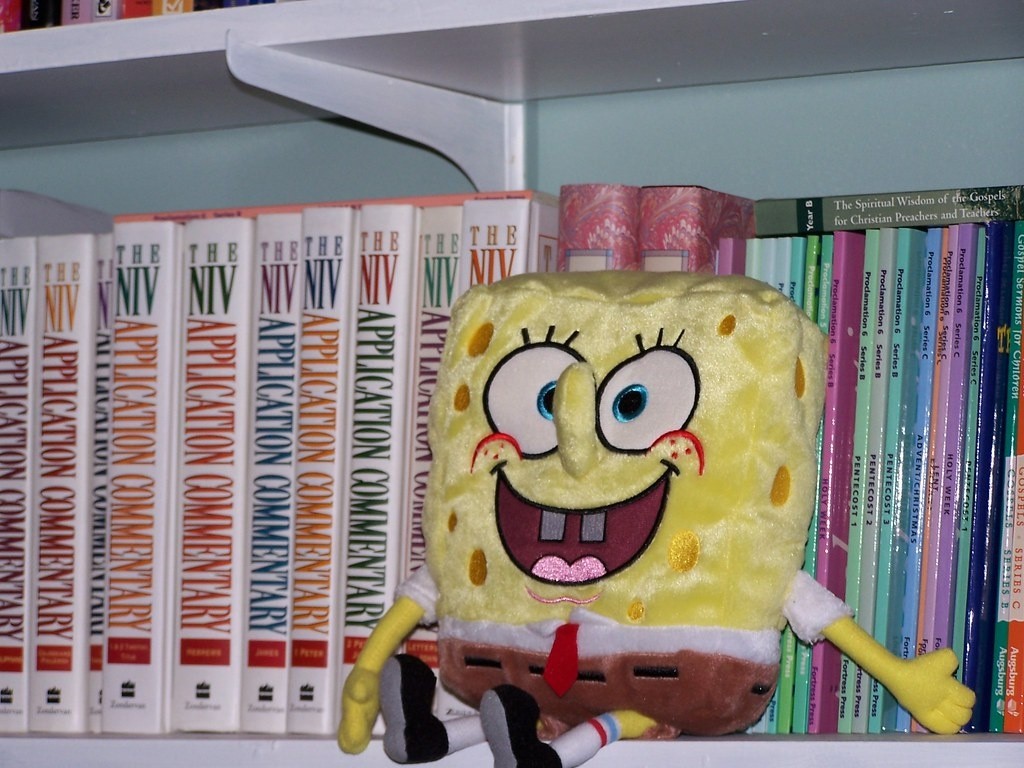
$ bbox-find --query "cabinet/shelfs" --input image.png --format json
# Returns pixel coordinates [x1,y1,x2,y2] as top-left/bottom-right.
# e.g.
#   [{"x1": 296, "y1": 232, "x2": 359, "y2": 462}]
[{"x1": 1, "y1": 0, "x2": 1024, "y2": 768}]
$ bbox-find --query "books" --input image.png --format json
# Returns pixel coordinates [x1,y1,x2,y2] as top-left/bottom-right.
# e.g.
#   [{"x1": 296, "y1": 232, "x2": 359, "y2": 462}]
[{"x1": 0, "y1": 1, "x2": 1021, "y2": 741}]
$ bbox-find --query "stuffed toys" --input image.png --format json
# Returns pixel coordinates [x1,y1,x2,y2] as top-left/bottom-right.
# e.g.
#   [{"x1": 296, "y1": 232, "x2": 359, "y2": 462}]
[{"x1": 336, "y1": 271, "x2": 977, "y2": 768}]
